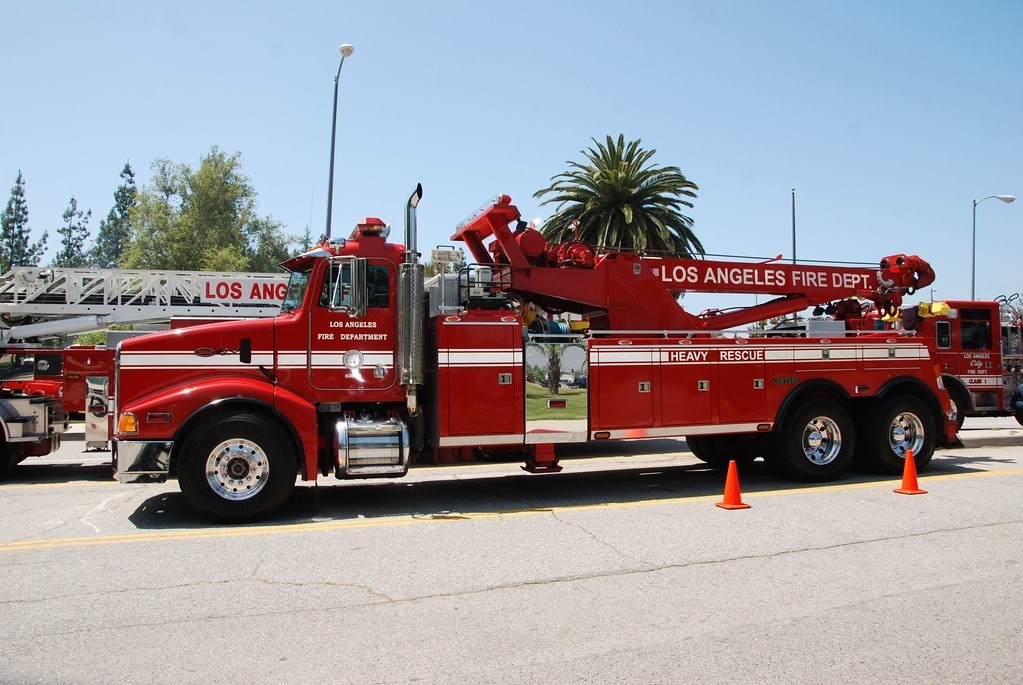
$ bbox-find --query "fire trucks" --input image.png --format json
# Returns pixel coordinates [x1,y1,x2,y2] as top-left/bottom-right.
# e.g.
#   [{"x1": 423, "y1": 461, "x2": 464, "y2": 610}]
[
  {"x1": 0, "y1": 266, "x2": 292, "y2": 479},
  {"x1": 107, "y1": 182, "x2": 1023, "y2": 525}
]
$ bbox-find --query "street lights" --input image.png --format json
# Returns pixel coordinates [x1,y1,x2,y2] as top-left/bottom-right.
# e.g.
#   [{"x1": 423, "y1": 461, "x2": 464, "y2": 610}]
[
  {"x1": 970, "y1": 194, "x2": 1016, "y2": 302},
  {"x1": 321, "y1": 43, "x2": 356, "y2": 246}
]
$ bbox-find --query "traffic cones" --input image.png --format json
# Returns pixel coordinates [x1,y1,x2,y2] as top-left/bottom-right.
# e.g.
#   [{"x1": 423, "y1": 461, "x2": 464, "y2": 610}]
[
  {"x1": 714, "y1": 460, "x2": 752, "y2": 510},
  {"x1": 891, "y1": 450, "x2": 929, "y2": 494}
]
